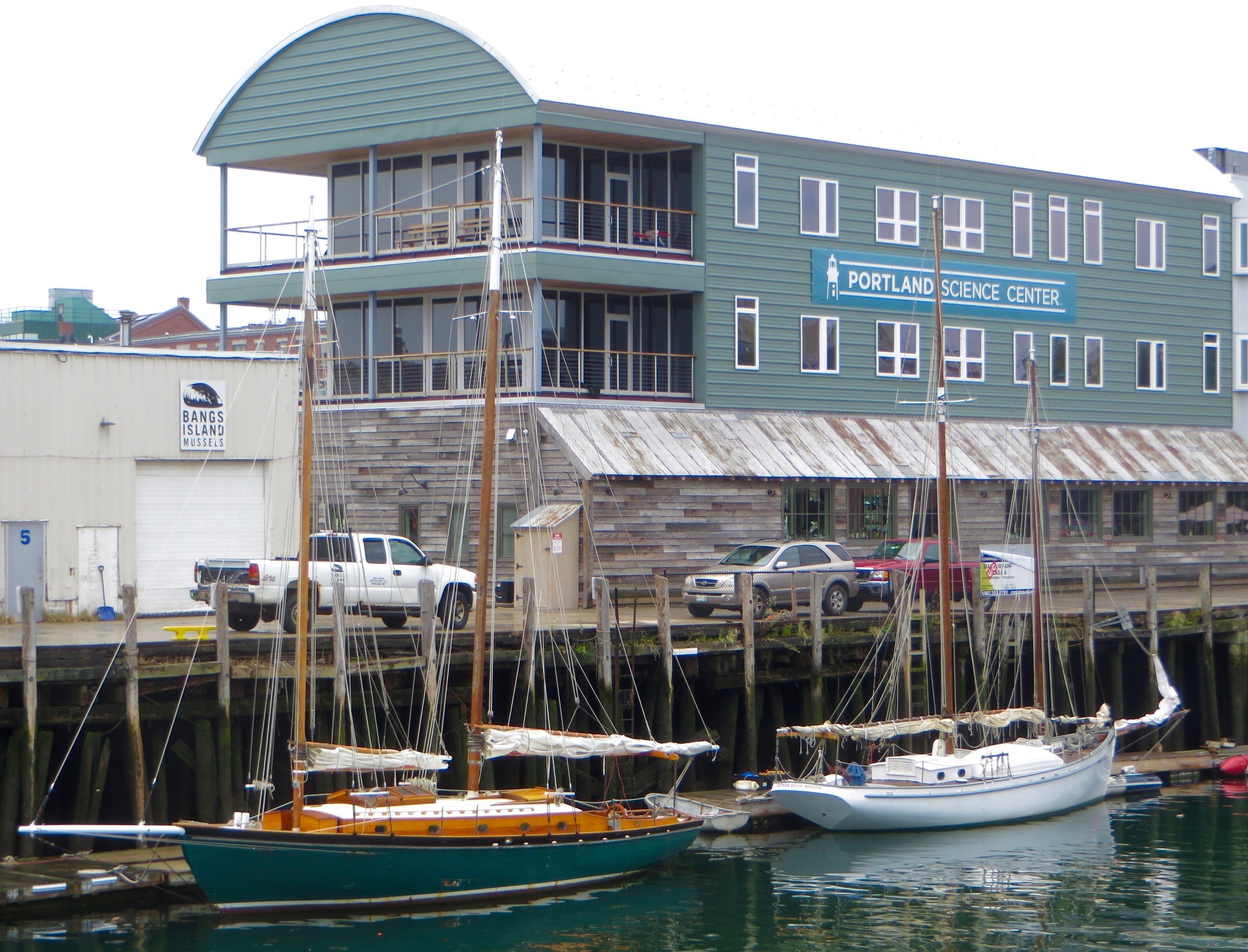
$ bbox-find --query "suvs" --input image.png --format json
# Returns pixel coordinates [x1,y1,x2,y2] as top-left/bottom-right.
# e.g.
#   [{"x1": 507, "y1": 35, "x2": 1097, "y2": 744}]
[{"x1": 682, "y1": 536, "x2": 861, "y2": 620}]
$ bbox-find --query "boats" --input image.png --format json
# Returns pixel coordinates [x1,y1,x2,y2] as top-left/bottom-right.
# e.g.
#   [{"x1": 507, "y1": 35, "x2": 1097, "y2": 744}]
[{"x1": 644, "y1": 792, "x2": 752, "y2": 836}]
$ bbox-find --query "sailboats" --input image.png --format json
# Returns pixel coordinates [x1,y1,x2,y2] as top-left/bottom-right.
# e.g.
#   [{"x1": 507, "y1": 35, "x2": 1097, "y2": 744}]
[
  {"x1": 731, "y1": 205, "x2": 1191, "y2": 838},
  {"x1": 14, "y1": 110, "x2": 724, "y2": 928}
]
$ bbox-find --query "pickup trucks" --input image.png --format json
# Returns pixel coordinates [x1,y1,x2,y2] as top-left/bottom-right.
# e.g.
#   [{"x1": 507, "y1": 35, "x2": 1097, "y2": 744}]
[
  {"x1": 845, "y1": 539, "x2": 997, "y2": 614},
  {"x1": 189, "y1": 529, "x2": 477, "y2": 635}
]
